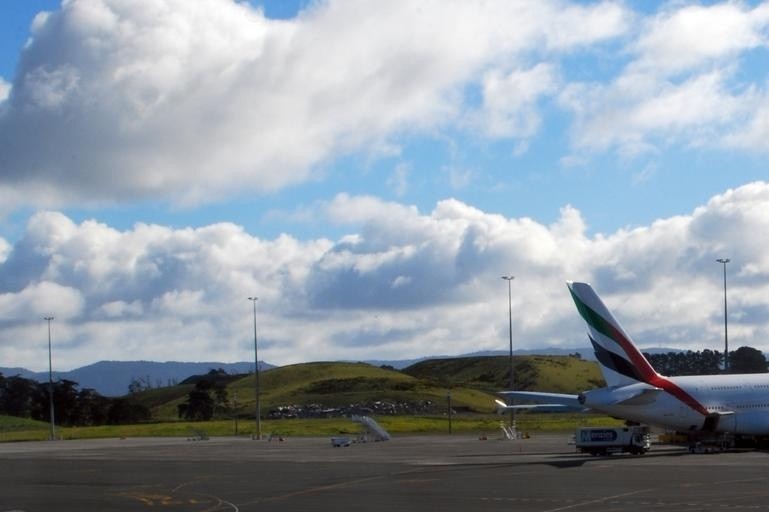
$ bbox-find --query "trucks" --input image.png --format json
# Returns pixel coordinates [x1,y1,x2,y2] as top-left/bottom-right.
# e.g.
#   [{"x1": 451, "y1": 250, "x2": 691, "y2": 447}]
[{"x1": 573, "y1": 424, "x2": 652, "y2": 457}]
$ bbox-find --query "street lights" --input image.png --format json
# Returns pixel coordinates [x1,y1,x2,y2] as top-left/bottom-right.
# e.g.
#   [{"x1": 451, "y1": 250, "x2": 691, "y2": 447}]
[
  {"x1": 501, "y1": 274, "x2": 517, "y2": 432},
  {"x1": 716, "y1": 258, "x2": 733, "y2": 373},
  {"x1": 43, "y1": 315, "x2": 58, "y2": 440},
  {"x1": 248, "y1": 295, "x2": 264, "y2": 440}
]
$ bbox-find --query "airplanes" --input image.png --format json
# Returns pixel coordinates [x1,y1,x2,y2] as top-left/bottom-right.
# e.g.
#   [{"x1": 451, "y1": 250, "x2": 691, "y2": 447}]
[{"x1": 495, "y1": 279, "x2": 769, "y2": 434}]
